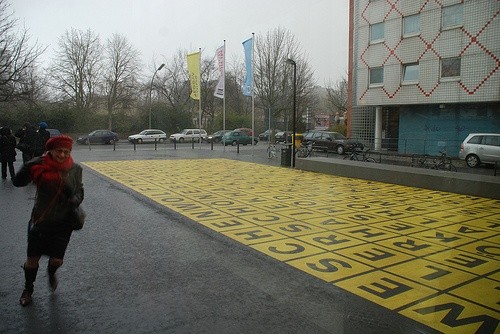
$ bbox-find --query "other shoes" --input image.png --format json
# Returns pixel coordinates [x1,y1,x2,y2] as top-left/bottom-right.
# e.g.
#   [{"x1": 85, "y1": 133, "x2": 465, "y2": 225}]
[
  {"x1": 1, "y1": 176, "x2": 7, "y2": 179},
  {"x1": 11, "y1": 177, "x2": 15, "y2": 180}
]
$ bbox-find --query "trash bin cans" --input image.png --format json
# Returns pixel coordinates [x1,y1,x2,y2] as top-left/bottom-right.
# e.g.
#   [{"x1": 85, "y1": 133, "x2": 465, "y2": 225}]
[{"x1": 280, "y1": 143, "x2": 292, "y2": 167}]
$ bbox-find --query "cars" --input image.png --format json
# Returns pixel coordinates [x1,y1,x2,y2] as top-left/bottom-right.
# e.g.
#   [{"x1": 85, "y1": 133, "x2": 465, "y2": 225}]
[
  {"x1": 259, "y1": 128, "x2": 303, "y2": 149},
  {"x1": 128, "y1": 129, "x2": 167, "y2": 144},
  {"x1": 300, "y1": 130, "x2": 364, "y2": 155},
  {"x1": 207, "y1": 128, "x2": 258, "y2": 146},
  {"x1": 36, "y1": 128, "x2": 62, "y2": 139},
  {"x1": 77, "y1": 129, "x2": 119, "y2": 146}
]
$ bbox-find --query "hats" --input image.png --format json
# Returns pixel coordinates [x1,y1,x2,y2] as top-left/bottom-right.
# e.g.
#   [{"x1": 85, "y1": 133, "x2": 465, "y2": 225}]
[
  {"x1": 44, "y1": 136, "x2": 73, "y2": 151},
  {"x1": 39, "y1": 122, "x2": 48, "y2": 128}
]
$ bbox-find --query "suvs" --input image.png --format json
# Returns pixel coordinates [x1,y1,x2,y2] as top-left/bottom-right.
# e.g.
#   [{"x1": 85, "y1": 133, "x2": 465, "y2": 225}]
[
  {"x1": 170, "y1": 128, "x2": 207, "y2": 143},
  {"x1": 459, "y1": 133, "x2": 500, "y2": 168}
]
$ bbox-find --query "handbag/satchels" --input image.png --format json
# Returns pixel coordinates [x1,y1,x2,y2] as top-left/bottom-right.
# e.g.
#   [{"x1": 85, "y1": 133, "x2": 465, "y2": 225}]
[
  {"x1": 70, "y1": 204, "x2": 86, "y2": 231},
  {"x1": 16, "y1": 143, "x2": 25, "y2": 150}
]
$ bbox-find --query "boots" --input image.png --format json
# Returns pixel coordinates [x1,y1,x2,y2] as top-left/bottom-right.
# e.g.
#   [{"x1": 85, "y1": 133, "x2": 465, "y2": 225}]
[
  {"x1": 47, "y1": 257, "x2": 63, "y2": 288},
  {"x1": 20, "y1": 263, "x2": 39, "y2": 306}
]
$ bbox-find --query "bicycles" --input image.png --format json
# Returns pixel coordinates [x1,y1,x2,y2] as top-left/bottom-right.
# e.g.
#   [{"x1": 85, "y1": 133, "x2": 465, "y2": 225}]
[
  {"x1": 412, "y1": 152, "x2": 457, "y2": 173},
  {"x1": 349, "y1": 144, "x2": 376, "y2": 164}
]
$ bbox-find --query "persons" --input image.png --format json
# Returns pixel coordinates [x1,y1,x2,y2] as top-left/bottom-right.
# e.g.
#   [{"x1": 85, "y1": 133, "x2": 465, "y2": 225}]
[
  {"x1": 0, "y1": 123, "x2": 51, "y2": 180},
  {"x1": 12, "y1": 134, "x2": 84, "y2": 307}
]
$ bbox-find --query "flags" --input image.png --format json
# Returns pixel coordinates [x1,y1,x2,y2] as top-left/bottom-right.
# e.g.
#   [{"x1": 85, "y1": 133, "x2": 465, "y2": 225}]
[
  {"x1": 242, "y1": 39, "x2": 253, "y2": 96},
  {"x1": 187, "y1": 51, "x2": 200, "y2": 100},
  {"x1": 213, "y1": 45, "x2": 225, "y2": 99}
]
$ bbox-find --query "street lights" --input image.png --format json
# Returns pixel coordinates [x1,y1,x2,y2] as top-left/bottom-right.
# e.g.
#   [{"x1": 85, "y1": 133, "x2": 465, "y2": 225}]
[
  {"x1": 149, "y1": 64, "x2": 165, "y2": 129},
  {"x1": 285, "y1": 58, "x2": 296, "y2": 167}
]
